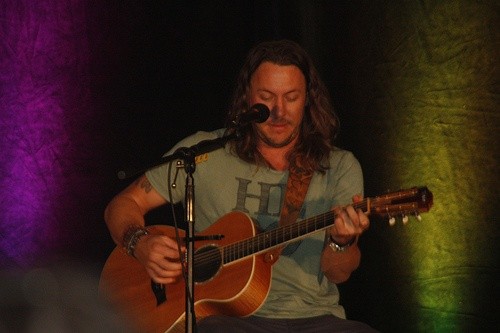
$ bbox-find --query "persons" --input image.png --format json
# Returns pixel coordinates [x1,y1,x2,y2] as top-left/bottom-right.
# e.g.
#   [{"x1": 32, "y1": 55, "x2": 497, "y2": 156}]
[{"x1": 105, "y1": 40, "x2": 372, "y2": 333}]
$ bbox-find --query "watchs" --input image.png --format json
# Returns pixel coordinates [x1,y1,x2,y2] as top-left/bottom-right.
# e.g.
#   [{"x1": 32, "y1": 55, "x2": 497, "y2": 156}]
[{"x1": 327, "y1": 232, "x2": 356, "y2": 253}]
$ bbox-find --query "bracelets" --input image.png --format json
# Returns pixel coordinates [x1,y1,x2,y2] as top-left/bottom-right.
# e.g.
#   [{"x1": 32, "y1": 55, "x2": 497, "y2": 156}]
[{"x1": 121, "y1": 225, "x2": 150, "y2": 259}]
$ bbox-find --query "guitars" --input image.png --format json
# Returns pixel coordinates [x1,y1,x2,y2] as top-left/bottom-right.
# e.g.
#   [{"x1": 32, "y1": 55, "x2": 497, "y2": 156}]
[{"x1": 97, "y1": 185, "x2": 434, "y2": 332}]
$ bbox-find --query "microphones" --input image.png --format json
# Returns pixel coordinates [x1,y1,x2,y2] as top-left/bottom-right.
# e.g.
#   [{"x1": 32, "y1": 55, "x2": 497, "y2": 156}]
[{"x1": 231, "y1": 103, "x2": 270, "y2": 129}]
